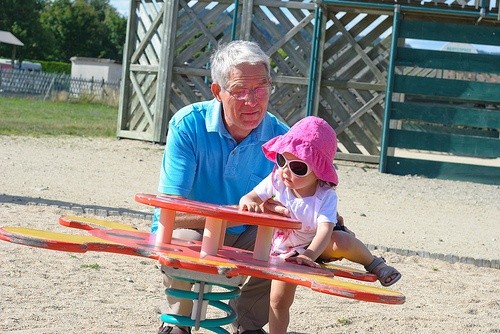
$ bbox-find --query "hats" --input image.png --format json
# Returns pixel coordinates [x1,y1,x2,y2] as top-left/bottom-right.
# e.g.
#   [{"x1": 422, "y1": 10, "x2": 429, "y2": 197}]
[{"x1": 261, "y1": 115, "x2": 338, "y2": 186}]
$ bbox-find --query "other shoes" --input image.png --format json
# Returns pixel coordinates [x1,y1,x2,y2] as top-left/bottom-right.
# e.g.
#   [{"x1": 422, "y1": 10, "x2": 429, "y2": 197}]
[{"x1": 225, "y1": 304, "x2": 267, "y2": 334}]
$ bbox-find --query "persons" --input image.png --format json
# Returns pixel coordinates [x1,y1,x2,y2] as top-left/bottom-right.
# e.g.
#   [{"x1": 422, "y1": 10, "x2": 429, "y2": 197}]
[
  {"x1": 152, "y1": 40, "x2": 343, "y2": 334},
  {"x1": 239, "y1": 116, "x2": 401, "y2": 334}
]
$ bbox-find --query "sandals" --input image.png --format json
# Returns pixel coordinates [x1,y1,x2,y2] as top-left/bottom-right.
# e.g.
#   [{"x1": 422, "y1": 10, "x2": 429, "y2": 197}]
[{"x1": 365, "y1": 252, "x2": 401, "y2": 287}]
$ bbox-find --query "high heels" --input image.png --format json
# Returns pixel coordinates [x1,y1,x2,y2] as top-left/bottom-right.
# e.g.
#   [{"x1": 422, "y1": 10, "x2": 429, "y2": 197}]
[{"x1": 159, "y1": 321, "x2": 192, "y2": 334}]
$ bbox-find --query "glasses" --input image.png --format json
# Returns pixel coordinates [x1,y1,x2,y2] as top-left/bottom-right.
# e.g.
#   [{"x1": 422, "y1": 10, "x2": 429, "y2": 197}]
[
  {"x1": 222, "y1": 75, "x2": 274, "y2": 101},
  {"x1": 274, "y1": 152, "x2": 313, "y2": 178}
]
